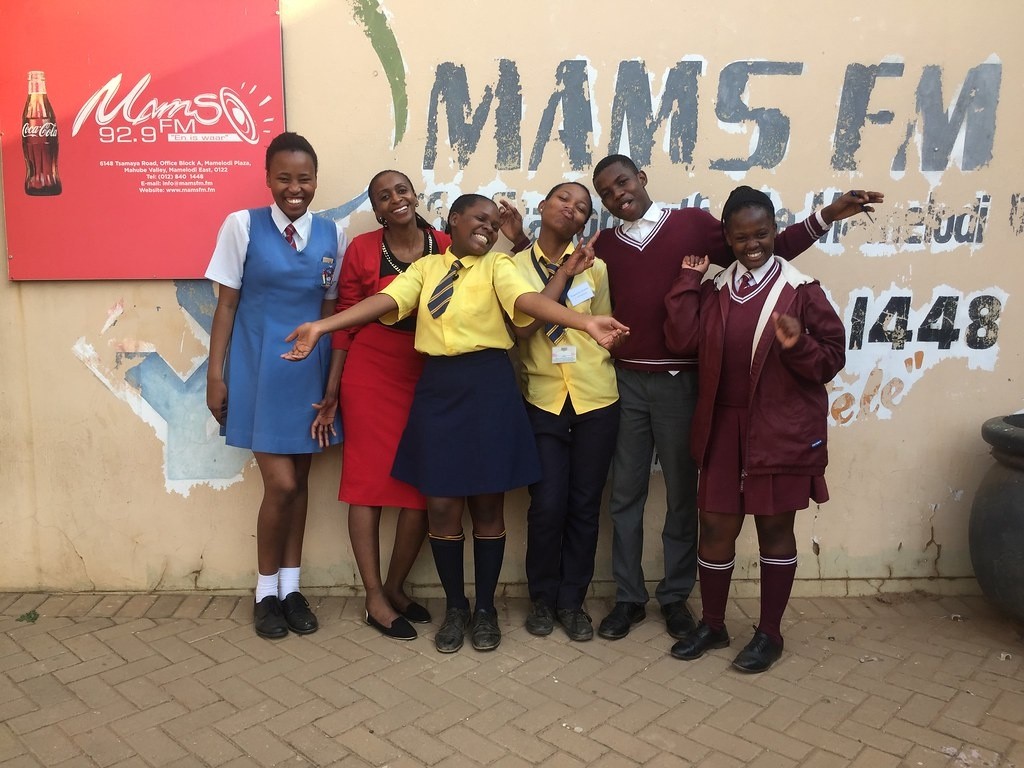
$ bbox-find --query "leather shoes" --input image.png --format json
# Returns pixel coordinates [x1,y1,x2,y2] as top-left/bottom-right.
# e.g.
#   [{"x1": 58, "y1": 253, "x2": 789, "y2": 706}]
[
  {"x1": 660, "y1": 601, "x2": 696, "y2": 639},
  {"x1": 253, "y1": 595, "x2": 289, "y2": 638},
  {"x1": 671, "y1": 619, "x2": 729, "y2": 659},
  {"x1": 733, "y1": 624, "x2": 782, "y2": 672},
  {"x1": 597, "y1": 601, "x2": 646, "y2": 639},
  {"x1": 282, "y1": 592, "x2": 318, "y2": 633}
]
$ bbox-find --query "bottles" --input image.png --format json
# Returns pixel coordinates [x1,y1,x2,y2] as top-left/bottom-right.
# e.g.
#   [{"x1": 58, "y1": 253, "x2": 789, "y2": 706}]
[{"x1": 21, "y1": 71, "x2": 62, "y2": 196}]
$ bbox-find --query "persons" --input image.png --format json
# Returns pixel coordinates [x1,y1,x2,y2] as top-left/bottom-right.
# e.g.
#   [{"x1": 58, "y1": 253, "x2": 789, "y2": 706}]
[
  {"x1": 662, "y1": 186, "x2": 849, "y2": 671},
  {"x1": 281, "y1": 192, "x2": 630, "y2": 651},
  {"x1": 504, "y1": 183, "x2": 621, "y2": 641},
  {"x1": 311, "y1": 171, "x2": 453, "y2": 640},
  {"x1": 204, "y1": 131, "x2": 348, "y2": 638},
  {"x1": 499, "y1": 155, "x2": 883, "y2": 651}
]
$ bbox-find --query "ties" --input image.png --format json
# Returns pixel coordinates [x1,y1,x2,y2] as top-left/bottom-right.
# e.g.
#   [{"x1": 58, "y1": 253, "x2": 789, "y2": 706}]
[
  {"x1": 739, "y1": 271, "x2": 753, "y2": 292},
  {"x1": 427, "y1": 260, "x2": 463, "y2": 319},
  {"x1": 283, "y1": 224, "x2": 296, "y2": 252},
  {"x1": 540, "y1": 254, "x2": 569, "y2": 346}
]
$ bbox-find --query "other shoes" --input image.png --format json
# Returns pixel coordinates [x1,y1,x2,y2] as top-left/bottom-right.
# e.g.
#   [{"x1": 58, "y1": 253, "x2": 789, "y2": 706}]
[
  {"x1": 389, "y1": 600, "x2": 432, "y2": 624},
  {"x1": 363, "y1": 607, "x2": 417, "y2": 640}
]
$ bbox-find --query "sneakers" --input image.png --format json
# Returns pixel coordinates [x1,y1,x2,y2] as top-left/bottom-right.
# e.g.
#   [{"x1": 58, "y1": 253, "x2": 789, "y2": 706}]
[
  {"x1": 554, "y1": 604, "x2": 593, "y2": 641},
  {"x1": 470, "y1": 605, "x2": 502, "y2": 649},
  {"x1": 525, "y1": 602, "x2": 553, "y2": 635},
  {"x1": 435, "y1": 597, "x2": 472, "y2": 652}
]
